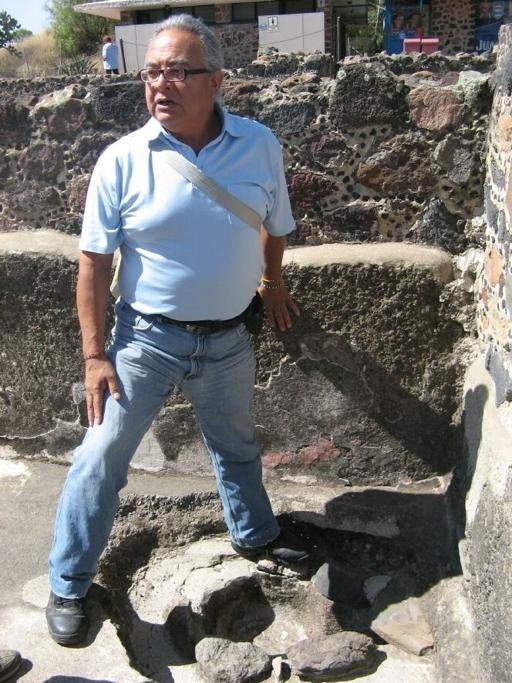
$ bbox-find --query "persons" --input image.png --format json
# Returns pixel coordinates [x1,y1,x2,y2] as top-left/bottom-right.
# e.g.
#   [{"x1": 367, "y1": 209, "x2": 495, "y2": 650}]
[
  {"x1": 405, "y1": 13, "x2": 423, "y2": 29},
  {"x1": 41, "y1": 10, "x2": 300, "y2": 643},
  {"x1": 97, "y1": 34, "x2": 119, "y2": 75},
  {"x1": 391, "y1": 11, "x2": 406, "y2": 32}
]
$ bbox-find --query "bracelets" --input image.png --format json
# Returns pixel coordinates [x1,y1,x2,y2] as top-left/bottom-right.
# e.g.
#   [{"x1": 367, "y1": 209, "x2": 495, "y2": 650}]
[
  {"x1": 260, "y1": 274, "x2": 282, "y2": 289},
  {"x1": 80, "y1": 351, "x2": 104, "y2": 362}
]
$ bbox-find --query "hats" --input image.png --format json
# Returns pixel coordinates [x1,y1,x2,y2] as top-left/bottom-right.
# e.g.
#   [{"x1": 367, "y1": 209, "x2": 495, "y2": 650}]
[{"x1": 103, "y1": 36, "x2": 111, "y2": 44}]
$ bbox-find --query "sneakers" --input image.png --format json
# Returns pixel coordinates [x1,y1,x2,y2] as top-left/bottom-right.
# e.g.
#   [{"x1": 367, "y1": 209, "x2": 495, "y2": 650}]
[
  {"x1": 47, "y1": 590, "x2": 89, "y2": 644},
  {"x1": 0, "y1": 650, "x2": 21, "y2": 681},
  {"x1": 230, "y1": 529, "x2": 316, "y2": 562}
]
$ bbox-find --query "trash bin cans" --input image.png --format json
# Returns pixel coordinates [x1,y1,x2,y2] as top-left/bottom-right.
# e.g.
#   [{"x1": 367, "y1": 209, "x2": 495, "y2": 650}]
[{"x1": 403, "y1": 37, "x2": 440, "y2": 54}]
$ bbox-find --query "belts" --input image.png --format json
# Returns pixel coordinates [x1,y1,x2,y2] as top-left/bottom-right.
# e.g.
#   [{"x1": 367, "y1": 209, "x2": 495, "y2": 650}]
[{"x1": 154, "y1": 315, "x2": 243, "y2": 335}]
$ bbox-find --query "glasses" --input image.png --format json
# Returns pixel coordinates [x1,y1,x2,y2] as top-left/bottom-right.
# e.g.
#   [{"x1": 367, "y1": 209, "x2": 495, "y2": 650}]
[{"x1": 139, "y1": 66, "x2": 211, "y2": 82}]
